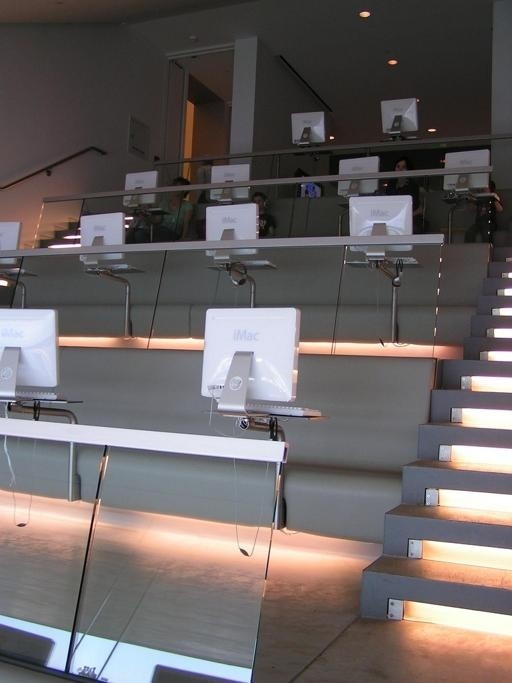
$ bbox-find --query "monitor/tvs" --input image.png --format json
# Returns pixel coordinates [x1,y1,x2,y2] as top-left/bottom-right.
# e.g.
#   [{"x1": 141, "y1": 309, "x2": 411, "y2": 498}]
[
  {"x1": 200, "y1": 307, "x2": 302, "y2": 419},
  {"x1": 205, "y1": 164, "x2": 260, "y2": 262},
  {"x1": 349, "y1": 195, "x2": 414, "y2": 258},
  {"x1": 0, "y1": 221, "x2": 22, "y2": 265},
  {"x1": 377, "y1": 97, "x2": 419, "y2": 142},
  {"x1": 443, "y1": 149, "x2": 490, "y2": 192},
  {"x1": 337, "y1": 156, "x2": 380, "y2": 196},
  {"x1": 79, "y1": 212, "x2": 125, "y2": 261},
  {"x1": 291, "y1": 111, "x2": 326, "y2": 146},
  {"x1": 0, "y1": 308, "x2": 61, "y2": 403},
  {"x1": 123, "y1": 171, "x2": 159, "y2": 208}
]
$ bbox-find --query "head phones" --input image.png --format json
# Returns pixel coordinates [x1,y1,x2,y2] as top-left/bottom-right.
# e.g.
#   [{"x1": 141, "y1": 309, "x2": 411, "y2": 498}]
[
  {"x1": 391, "y1": 258, "x2": 404, "y2": 288},
  {"x1": 264, "y1": 199, "x2": 272, "y2": 209},
  {"x1": 229, "y1": 263, "x2": 248, "y2": 288}
]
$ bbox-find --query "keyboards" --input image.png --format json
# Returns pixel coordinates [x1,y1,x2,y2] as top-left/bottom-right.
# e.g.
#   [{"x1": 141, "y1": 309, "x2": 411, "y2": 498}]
[
  {"x1": 245, "y1": 403, "x2": 322, "y2": 418},
  {"x1": 16, "y1": 392, "x2": 67, "y2": 401}
]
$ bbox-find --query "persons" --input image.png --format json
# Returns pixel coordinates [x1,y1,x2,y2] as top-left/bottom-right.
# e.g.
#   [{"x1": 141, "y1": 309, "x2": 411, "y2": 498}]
[
  {"x1": 123, "y1": 174, "x2": 197, "y2": 242},
  {"x1": 385, "y1": 157, "x2": 424, "y2": 232},
  {"x1": 251, "y1": 190, "x2": 278, "y2": 238},
  {"x1": 462, "y1": 178, "x2": 504, "y2": 242}
]
